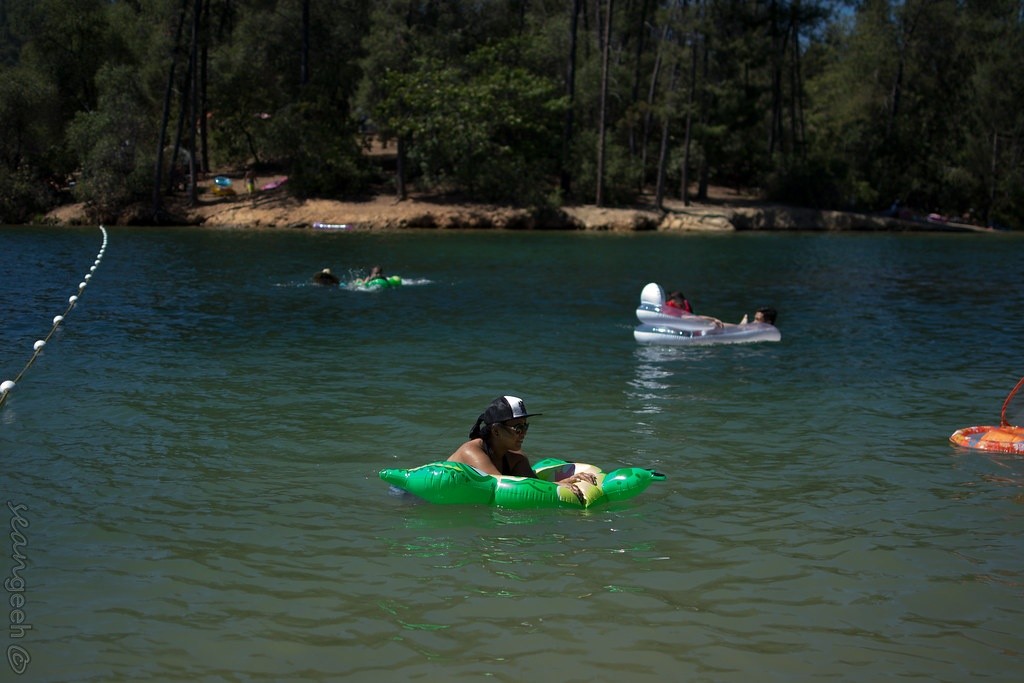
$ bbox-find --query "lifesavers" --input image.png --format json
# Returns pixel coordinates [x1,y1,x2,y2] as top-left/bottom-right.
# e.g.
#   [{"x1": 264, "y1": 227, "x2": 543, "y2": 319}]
[
  {"x1": 949, "y1": 426, "x2": 1024, "y2": 453},
  {"x1": 633, "y1": 283, "x2": 781, "y2": 346},
  {"x1": 313, "y1": 222, "x2": 354, "y2": 230},
  {"x1": 376, "y1": 458, "x2": 665, "y2": 508},
  {"x1": 338, "y1": 275, "x2": 401, "y2": 291},
  {"x1": 216, "y1": 175, "x2": 232, "y2": 186}
]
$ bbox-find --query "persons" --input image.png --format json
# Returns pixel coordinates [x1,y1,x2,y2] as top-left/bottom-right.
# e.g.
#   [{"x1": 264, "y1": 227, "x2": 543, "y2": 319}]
[
  {"x1": 365, "y1": 266, "x2": 389, "y2": 284},
  {"x1": 446, "y1": 395, "x2": 597, "y2": 506},
  {"x1": 663, "y1": 293, "x2": 776, "y2": 336}
]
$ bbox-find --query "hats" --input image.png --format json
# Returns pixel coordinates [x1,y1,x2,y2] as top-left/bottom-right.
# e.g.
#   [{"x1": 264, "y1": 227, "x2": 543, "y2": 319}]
[{"x1": 484, "y1": 396, "x2": 542, "y2": 424}]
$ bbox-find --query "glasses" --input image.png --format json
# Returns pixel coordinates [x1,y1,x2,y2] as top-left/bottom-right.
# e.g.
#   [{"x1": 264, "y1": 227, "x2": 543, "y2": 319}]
[{"x1": 498, "y1": 422, "x2": 531, "y2": 433}]
